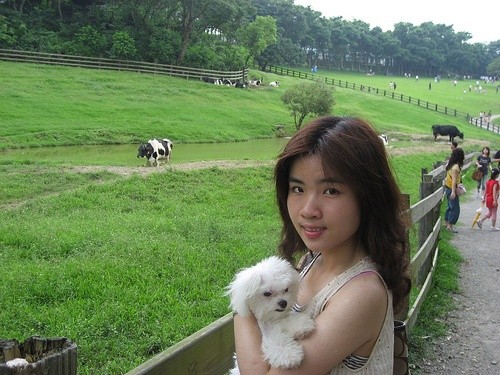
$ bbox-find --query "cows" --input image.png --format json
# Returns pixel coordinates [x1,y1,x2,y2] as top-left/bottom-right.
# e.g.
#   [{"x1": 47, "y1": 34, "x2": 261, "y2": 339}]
[
  {"x1": 431, "y1": 125, "x2": 464, "y2": 142},
  {"x1": 213, "y1": 76, "x2": 262, "y2": 88},
  {"x1": 137, "y1": 138, "x2": 173, "y2": 167}
]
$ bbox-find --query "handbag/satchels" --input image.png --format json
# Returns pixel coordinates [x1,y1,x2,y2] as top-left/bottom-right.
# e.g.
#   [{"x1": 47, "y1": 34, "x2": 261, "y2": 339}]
[
  {"x1": 456, "y1": 183, "x2": 466, "y2": 195},
  {"x1": 300, "y1": 251, "x2": 410, "y2": 375},
  {"x1": 472, "y1": 169, "x2": 483, "y2": 181}
]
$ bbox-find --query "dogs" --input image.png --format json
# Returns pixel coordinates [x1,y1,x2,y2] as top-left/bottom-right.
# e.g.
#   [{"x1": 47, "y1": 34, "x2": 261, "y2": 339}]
[{"x1": 222, "y1": 254, "x2": 318, "y2": 375}]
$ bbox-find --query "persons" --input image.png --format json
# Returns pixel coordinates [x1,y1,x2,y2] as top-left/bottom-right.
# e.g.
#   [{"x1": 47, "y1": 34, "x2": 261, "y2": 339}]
[
  {"x1": 220, "y1": 113, "x2": 411, "y2": 375},
  {"x1": 491, "y1": 150, "x2": 500, "y2": 168},
  {"x1": 475, "y1": 168, "x2": 500, "y2": 231},
  {"x1": 442, "y1": 148, "x2": 465, "y2": 232},
  {"x1": 310, "y1": 63, "x2": 500, "y2": 123},
  {"x1": 471, "y1": 147, "x2": 493, "y2": 202},
  {"x1": 451, "y1": 141, "x2": 457, "y2": 150}
]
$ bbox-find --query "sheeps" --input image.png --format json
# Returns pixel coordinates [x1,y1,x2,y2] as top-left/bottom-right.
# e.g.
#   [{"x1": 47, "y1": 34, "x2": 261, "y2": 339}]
[{"x1": 269, "y1": 82, "x2": 280, "y2": 87}]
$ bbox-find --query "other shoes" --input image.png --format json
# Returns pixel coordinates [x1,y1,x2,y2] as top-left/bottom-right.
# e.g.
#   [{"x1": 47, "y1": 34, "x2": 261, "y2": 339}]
[{"x1": 443, "y1": 192, "x2": 500, "y2": 232}]
